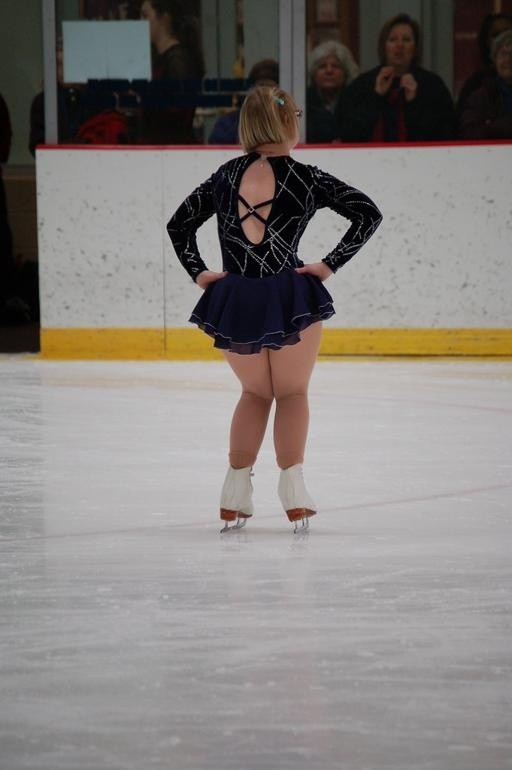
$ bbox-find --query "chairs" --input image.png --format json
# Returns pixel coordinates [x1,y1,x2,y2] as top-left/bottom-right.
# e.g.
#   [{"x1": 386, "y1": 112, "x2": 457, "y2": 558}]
[{"x1": 60, "y1": 78, "x2": 249, "y2": 143}]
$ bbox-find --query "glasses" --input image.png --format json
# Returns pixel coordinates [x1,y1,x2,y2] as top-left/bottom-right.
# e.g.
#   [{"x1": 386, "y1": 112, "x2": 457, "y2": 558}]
[{"x1": 294, "y1": 109, "x2": 303, "y2": 117}]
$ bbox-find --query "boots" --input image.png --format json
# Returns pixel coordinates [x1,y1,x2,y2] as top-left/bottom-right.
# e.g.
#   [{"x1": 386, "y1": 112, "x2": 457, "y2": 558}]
[
  {"x1": 218, "y1": 462, "x2": 255, "y2": 521},
  {"x1": 278, "y1": 463, "x2": 318, "y2": 522}
]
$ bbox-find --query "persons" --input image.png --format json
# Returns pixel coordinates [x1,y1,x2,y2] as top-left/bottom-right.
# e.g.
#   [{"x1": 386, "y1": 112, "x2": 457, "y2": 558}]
[
  {"x1": 163, "y1": 81, "x2": 387, "y2": 526},
  {"x1": 205, "y1": 56, "x2": 280, "y2": 146},
  {"x1": 336, "y1": 9, "x2": 461, "y2": 143},
  {"x1": 458, "y1": 11, "x2": 512, "y2": 105},
  {"x1": 1, "y1": 91, "x2": 16, "y2": 323},
  {"x1": 85, "y1": 0, "x2": 201, "y2": 145},
  {"x1": 305, "y1": 41, "x2": 360, "y2": 145},
  {"x1": 28, "y1": 42, "x2": 105, "y2": 159},
  {"x1": 459, "y1": 27, "x2": 512, "y2": 139}
]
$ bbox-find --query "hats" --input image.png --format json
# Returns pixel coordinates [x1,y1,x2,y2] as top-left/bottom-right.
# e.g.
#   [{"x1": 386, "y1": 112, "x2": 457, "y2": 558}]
[{"x1": 247, "y1": 58, "x2": 280, "y2": 82}]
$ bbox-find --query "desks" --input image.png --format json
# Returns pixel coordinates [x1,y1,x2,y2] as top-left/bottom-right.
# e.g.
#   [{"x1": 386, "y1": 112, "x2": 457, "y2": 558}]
[{"x1": 2, "y1": 163, "x2": 36, "y2": 262}]
